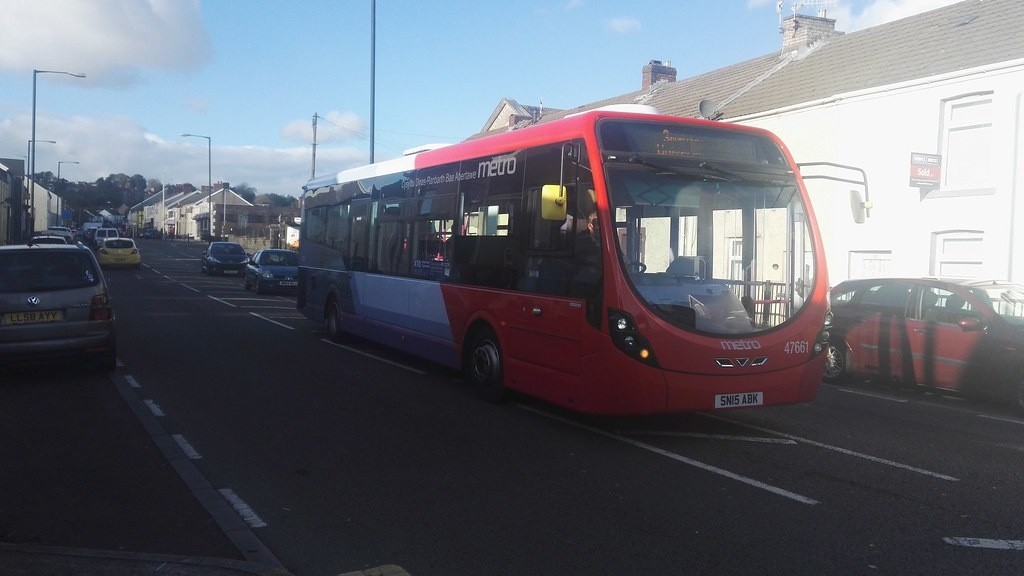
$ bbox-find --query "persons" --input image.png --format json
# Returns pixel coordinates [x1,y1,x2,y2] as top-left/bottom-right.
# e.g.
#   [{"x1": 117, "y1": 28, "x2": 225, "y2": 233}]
[{"x1": 575, "y1": 203, "x2": 638, "y2": 272}]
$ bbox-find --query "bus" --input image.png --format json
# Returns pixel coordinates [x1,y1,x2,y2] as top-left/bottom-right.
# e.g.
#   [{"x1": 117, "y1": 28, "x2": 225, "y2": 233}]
[{"x1": 293, "y1": 108, "x2": 872, "y2": 418}]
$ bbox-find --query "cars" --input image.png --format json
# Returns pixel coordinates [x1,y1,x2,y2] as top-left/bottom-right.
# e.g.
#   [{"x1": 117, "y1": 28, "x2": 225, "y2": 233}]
[
  {"x1": 139, "y1": 228, "x2": 161, "y2": 239},
  {"x1": 200, "y1": 240, "x2": 250, "y2": 277},
  {"x1": 96, "y1": 236, "x2": 141, "y2": 270},
  {"x1": 34, "y1": 224, "x2": 120, "y2": 250},
  {"x1": 243, "y1": 249, "x2": 301, "y2": 295}
]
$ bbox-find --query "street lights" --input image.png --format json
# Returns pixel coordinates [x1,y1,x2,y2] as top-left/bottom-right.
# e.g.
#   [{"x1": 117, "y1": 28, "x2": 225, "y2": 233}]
[
  {"x1": 24, "y1": 139, "x2": 56, "y2": 241},
  {"x1": 179, "y1": 132, "x2": 211, "y2": 246},
  {"x1": 30, "y1": 68, "x2": 86, "y2": 241},
  {"x1": 56, "y1": 161, "x2": 79, "y2": 226}
]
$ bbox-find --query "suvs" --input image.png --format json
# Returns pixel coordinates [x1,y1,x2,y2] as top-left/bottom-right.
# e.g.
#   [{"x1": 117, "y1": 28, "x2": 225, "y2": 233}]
[
  {"x1": 813, "y1": 276, "x2": 1024, "y2": 417},
  {"x1": 0, "y1": 241, "x2": 118, "y2": 374}
]
